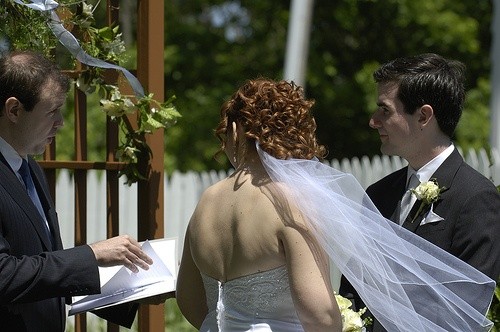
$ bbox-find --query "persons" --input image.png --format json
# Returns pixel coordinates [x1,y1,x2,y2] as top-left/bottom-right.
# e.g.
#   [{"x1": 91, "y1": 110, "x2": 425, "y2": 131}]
[
  {"x1": 0, "y1": 50, "x2": 154, "y2": 332},
  {"x1": 339, "y1": 53, "x2": 500, "y2": 332},
  {"x1": 176, "y1": 77, "x2": 342, "y2": 332}
]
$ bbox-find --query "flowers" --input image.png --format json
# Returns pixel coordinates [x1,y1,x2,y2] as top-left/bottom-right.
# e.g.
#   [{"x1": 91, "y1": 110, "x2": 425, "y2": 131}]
[
  {"x1": 0, "y1": 0, "x2": 183, "y2": 188},
  {"x1": 409, "y1": 177, "x2": 446, "y2": 223},
  {"x1": 333, "y1": 290, "x2": 373, "y2": 332}
]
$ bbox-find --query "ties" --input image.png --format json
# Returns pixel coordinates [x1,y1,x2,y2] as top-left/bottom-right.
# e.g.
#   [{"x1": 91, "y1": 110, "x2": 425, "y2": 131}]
[
  {"x1": 391, "y1": 176, "x2": 417, "y2": 225},
  {"x1": 19, "y1": 159, "x2": 45, "y2": 224}
]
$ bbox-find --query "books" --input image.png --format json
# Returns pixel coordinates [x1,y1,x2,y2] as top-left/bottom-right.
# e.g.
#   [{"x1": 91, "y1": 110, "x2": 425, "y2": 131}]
[{"x1": 68, "y1": 237, "x2": 179, "y2": 317}]
